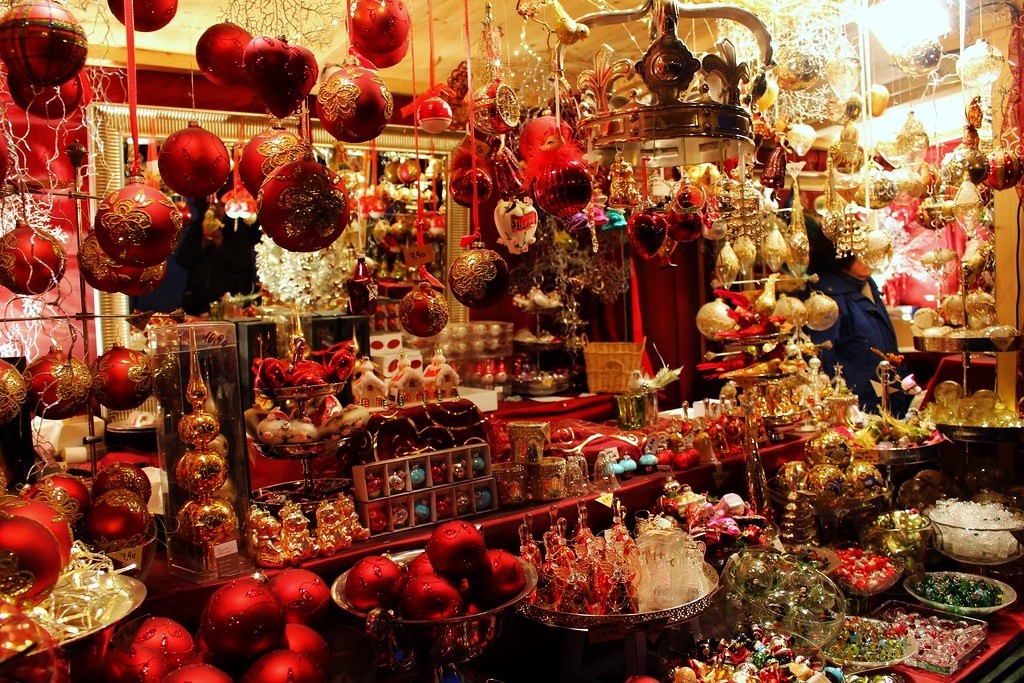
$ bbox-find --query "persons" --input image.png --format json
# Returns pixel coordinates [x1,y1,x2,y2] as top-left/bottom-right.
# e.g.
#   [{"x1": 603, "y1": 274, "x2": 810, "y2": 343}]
[{"x1": 800, "y1": 244, "x2": 900, "y2": 419}]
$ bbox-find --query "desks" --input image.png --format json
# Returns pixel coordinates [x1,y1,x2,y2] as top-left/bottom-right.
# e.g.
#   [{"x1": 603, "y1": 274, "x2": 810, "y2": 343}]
[{"x1": 125, "y1": 390, "x2": 816, "y2": 628}]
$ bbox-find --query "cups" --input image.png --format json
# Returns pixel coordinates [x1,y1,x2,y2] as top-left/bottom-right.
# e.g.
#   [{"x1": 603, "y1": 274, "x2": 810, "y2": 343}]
[{"x1": 491, "y1": 423, "x2": 565, "y2": 506}]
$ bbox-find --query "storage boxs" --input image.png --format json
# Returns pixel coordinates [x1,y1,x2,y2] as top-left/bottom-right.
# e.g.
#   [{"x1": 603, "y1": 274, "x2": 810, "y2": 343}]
[{"x1": 352, "y1": 443, "x2": 499, "y2": 538}]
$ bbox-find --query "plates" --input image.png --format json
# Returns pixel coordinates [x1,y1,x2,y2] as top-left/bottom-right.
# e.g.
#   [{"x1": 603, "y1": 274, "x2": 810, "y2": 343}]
[
  {"x1": 823, "y1": 616, "x2": 918, "y2": 668},
  {"x1": 904, "y1": 571, "x2": 1017, "y2": 618}
]
